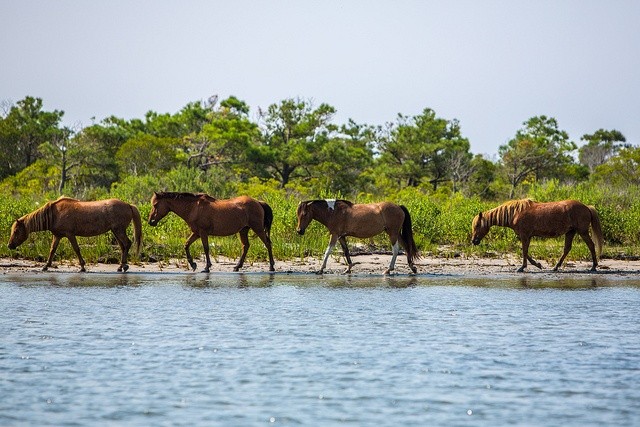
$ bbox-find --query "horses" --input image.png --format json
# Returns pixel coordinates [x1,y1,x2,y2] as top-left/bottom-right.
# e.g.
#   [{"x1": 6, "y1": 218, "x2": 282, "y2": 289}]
[
  {"x1": 296, "y1": 199, "x2": 423, "y2": 275},
  {"x1": 7, "y1": 197, "x2": 142, "y2": 273},
  {"x1": 471, "y1": 200, "x2": 603, "y2": 273},
  {"x1": 147, "y1": 190, "x2": 275, "y2": 273}
]
